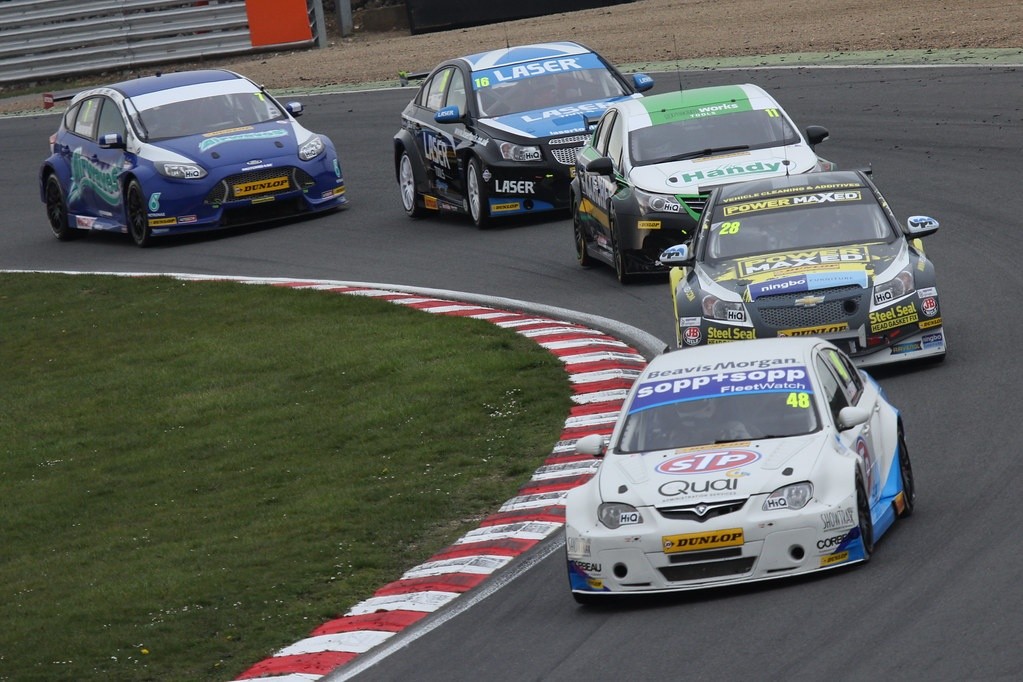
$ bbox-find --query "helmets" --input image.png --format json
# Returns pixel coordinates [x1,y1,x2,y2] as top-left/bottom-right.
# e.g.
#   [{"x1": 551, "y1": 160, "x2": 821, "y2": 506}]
[
  {"x1": 670, "y1": 398, "x2": 717, "y2": 435},
  {"x1": 641, "y1": 123, "x2": 674, "y2": 158},
  {"x1": 529, "y1": 75, "x2": 559, "y2": 101}
]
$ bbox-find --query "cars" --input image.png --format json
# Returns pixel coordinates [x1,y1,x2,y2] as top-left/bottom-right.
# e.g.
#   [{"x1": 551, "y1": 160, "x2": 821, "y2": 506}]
[
  {"x1": 39, "y1": 67, "x2": 350, "y2": 250},
  {"x1": 658, "y1": 167, "x2": 948, "y2": 373},
  {"x1": 394, "y1": 40, "x2": 655, "y2": 232},
  {"x1": 567, "y1": 83, "x2": 838, "y2": 288},
  {"x1": 564, "y1": 336, "x2": 917, "y2": 608}
]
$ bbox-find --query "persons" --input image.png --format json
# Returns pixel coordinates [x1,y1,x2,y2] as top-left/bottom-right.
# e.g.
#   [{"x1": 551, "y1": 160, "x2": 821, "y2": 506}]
[
  {"x1": 667, "y1": 397, "x2": 749, "y2": 447},
  {"x1": 528, "y1": 74, "x2": 559, "y2": 107},
  {"x1": 639, "y1": 127, "x2": 672, "y2": 161}
]
